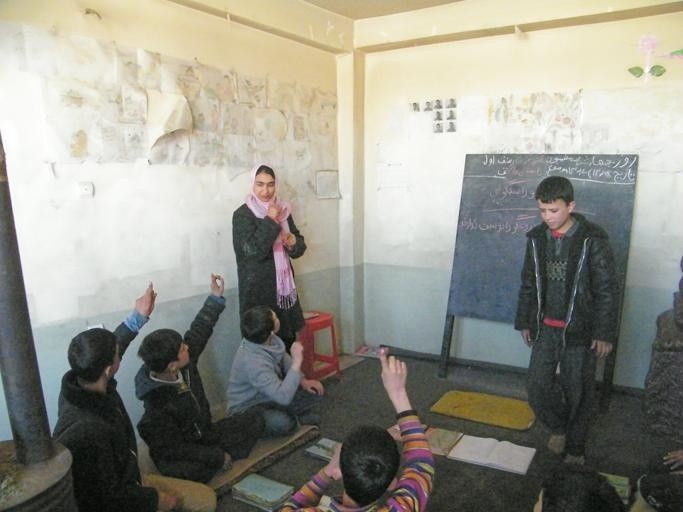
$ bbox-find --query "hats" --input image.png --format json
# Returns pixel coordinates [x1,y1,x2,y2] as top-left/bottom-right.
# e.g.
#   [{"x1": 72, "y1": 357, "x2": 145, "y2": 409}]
[{"x1": 634, "y1": 471, "x2": 682, "y2": 510}]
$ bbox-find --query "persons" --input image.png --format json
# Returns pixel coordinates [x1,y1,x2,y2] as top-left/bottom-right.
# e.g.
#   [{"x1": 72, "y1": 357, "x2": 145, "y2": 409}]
[
  {"x1": 134, "y1": 267, "x2": 263, "y2": 485},
  {"x1": 410, "y1": 98, "x2": 456, "y2": 135},
  {"x1": 629, "y1": 449, "x2": 681, "y2": 512},
  {"x1": 224, "y1": 305, "x2": 326, "y2": 439},
  {"x1": 280, "y1": 347, "x2": 436, "y2": 512},
  {"x1": 539, "y1": 462, "x2": 626, "y2": 512},
  {"x1": 51, "y1": 282, "x2": 217, "y2": 512},
  {"x1": 514, "y1": 174, "x2": 618, "y2": 467},
  {"x1": 639, "y1": 257, "x2": 682, "y2": 453},
  {"x1": 232, "y1": 161, "x2": 306, "y2": 352}
]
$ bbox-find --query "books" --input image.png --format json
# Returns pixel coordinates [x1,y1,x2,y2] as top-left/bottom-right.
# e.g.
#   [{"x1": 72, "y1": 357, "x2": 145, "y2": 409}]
[
  {"x1": 354, "y1": 345, "x2": 388, "y2": 360},
  {"x1": 424, "y1": 427, "x2": 462, "y2": 457},
  {"x1": 231, "y1": 473, "x2": 294, "y2": 512},
  {"x1": 304, "y1": 438, "x2": 338, "y2": 463},
  {"x1": 445, "y1": 434, "x2": 535, "y2": 476}
]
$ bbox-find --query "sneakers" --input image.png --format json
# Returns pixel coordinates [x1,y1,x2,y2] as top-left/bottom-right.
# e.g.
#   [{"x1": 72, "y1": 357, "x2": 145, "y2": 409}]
[
  {"x1": 546, "y1": 434, "x2": 565, "y2": 454},
  {"x1": 555, "y1": 454, "x2": 586, "y2": 481}
]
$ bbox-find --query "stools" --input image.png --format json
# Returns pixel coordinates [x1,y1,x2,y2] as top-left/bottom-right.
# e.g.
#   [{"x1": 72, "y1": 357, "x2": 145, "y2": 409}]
[{"x1": 299, "y1": 310, "x2": 343, "y2": 379}]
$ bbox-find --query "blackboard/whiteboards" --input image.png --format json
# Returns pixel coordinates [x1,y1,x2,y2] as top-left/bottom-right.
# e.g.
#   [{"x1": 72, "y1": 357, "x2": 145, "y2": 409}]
[{"x1": 447, "y1": 153, "x2": 639, "y2": 346}]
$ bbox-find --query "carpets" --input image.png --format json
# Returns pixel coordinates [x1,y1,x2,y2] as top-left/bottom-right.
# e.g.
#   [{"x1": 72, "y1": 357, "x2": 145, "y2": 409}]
[{"x1": 429, "y1": 390, "x2": 536, "y2": 431}]
